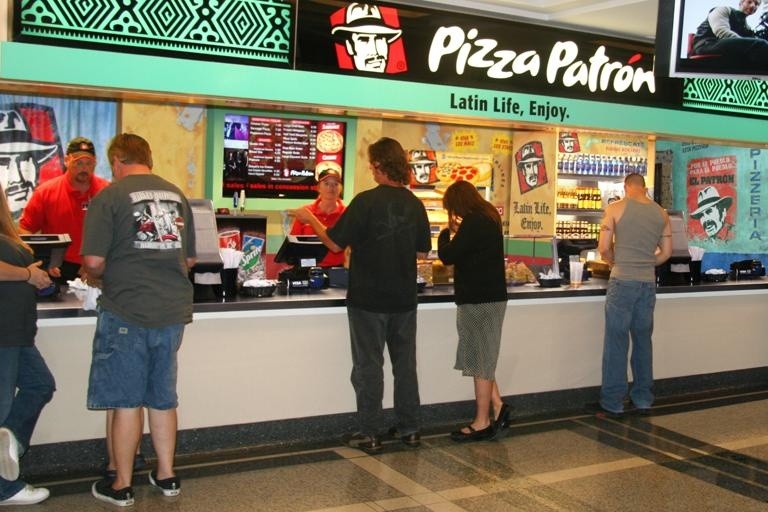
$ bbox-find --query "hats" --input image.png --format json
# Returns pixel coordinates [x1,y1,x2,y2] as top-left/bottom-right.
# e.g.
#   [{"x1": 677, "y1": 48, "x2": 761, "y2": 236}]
[
  {"x1": 318, "y1": 169, "x2": 341, "y2": 183},
  {"x1": 517, "y1": 144, "x2": 543, "y2": 169},
  {"x1": 66, "y1": 137, "x2": 96, "y2": 162},
  {"x1": 331, "y1": 2, "x2": 403, "y2": 44},
  {"x1": 689, "y1": 186, "x2": 733, "y2": 219},
  {"x1": 0, "y1": 109, "x2": 58, "y2": 165},
  {"x1": 407, "y1": 150, "x2": 436, "y2": 169},
  {"x1": 559, "y1": 133, "x2": 578, "y2": 144}
]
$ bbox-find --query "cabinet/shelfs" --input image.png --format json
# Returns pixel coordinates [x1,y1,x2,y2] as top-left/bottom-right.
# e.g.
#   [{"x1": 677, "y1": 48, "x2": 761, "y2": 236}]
[{"x1": 509, "y1": 131, "x2": 656, "y2": 241}]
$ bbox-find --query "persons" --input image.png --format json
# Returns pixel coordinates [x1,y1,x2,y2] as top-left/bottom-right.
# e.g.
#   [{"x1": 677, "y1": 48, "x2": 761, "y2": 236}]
[
  {"x1": 0, "y1": 108, "x2": 59, "y2": 213},
  {"x1": 689, "y1": 186, "x2": 736, "y2": 244},
  {"x1": 517, "y1": 144, "x2": 544, "y2": 188},
  {"x1": 331, "y1": 2, "x2": 403, "y2": 74},
  {"x1": 289, "y1": 160, "x2": 352, "y2": 270},
  {"x1": 17, "y1": 137, "x2": 112, "y2": 284},
  {"x1": 559, "y1": 132, "x2": 578, "y2": 153},
  {"x1": 407, "y1": 150, "x2": 436, "y2": 184},
  {"x1": 0, "y1": 183, "x2": 57, "y2": 506},
  {"x1": 438, "y1": 181, "x2": 510, "y2": 443},
  {"x1": 79, "y1": 133, "x2": 197, "y2": 507},
  {"x1": 285, "y1": 137, "x2": 432, "y2": 454},
  {"x1": 692, "y1": 0, "x2": 768, "y2": 55},
  {"x1": 597, "y1": 173, "x2": 673, "y2": 419}
]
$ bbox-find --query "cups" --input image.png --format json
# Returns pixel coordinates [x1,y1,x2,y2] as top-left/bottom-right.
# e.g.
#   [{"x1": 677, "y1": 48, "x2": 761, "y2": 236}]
[
  {"x1": 217, "y1": 230, "x2": 265, "y2": 272},
  {"x1": 569, "y1": 261, "x2": 584, "y2": 288},
  {"x1": 216, "y1": 268, "x2": 239, "y2": 298}
]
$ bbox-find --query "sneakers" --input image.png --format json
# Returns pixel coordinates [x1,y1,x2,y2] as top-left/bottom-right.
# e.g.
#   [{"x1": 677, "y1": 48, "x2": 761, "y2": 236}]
[
  {"x1": 91, "y1": 478, "x2": 136, "y2": 507},
  {"x1": 1, "y1": 426, "x2": 20, "y2": 481},
  {"x1": 148, "y1": 467, "x2": 181, "y2": 497},
  {"x1": 100, "y1": 462, "x2": 117, "y2": 477},
  {"x1": 1, "y1": 484, "x2": 51, "y2": 507},
  {"x1": 134, "y1": 454, "x2": 147, "y2": 470}
]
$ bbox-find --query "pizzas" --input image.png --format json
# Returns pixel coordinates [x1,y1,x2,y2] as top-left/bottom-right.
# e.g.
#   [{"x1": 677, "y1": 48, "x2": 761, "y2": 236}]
[
  {"x1": 474, "y1": 162, "x2": 492, "y2": 182},
  {"x1": 435, "y1": 161, "x2": 461, "y2": 183},
  {"x1": 451, "y1": 163, "x2": 479, "y2": 187}
]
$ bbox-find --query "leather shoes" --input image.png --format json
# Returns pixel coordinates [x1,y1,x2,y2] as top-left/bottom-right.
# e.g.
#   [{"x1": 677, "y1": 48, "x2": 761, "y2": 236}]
[
  {"x1": 389, "y1": 425, "x2": 421, "y2": 446},
  {"x1": 342, "y1": 431, "x2": 383, "y2": 453},
  {"x1": 451, "y1": 418, "x2": 497, "y2": 441},
  {"x1": 496, "y1": 403, "x2": 512, "y2": 438},
  {"x1": 637, "y1": 409, "x2": 649, "y2": 414},
  {"x1": 585, "y1": 400, "x2": 623, "y2": 418}
]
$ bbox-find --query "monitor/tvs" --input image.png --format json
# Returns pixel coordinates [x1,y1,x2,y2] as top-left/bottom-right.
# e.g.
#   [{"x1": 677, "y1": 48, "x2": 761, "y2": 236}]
[
  {"x1": 275, "y1": 235, "x2": 328, "y2": 272},
  {"x1": 655, "y1": 0, "x2": 768, "y2": 81}
]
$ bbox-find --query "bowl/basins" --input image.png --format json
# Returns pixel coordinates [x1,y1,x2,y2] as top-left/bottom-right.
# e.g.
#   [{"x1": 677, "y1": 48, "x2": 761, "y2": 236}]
[
  {"x1": 242, "y1": 285, "x2": 277, "y2": 298},
  {"x1": 536, "y1": 275, "x2": 563, "y2": 287},
  {"x1": 701, "y1": 271, "x2": 730, "y2": 282}
]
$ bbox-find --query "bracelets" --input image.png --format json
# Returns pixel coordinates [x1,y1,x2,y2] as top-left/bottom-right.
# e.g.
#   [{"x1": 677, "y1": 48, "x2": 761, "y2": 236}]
[{"x1": 25, "y1": 267, "x2": 31, "y2": 283}]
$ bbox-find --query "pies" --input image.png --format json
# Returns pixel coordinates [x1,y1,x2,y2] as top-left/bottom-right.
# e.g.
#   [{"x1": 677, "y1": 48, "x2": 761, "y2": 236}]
[
  {"x1": 317, "y1": 130, "x2": 344, "y2": 154},
  {"x1": 314, "y1": 161, "x2": 343, "y2": 181}
]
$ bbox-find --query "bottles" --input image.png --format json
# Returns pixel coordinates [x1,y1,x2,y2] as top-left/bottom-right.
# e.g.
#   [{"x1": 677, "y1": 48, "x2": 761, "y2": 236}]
[
  {"x1": 558, "y1": 153, "x2": 647, "y2": 176},
  {"x1": 556, "y1": 186, "x2": 602, "y2": 210},
  {"x1": 555, "y1": 220, "x2": 601, "y2": 241}
]
